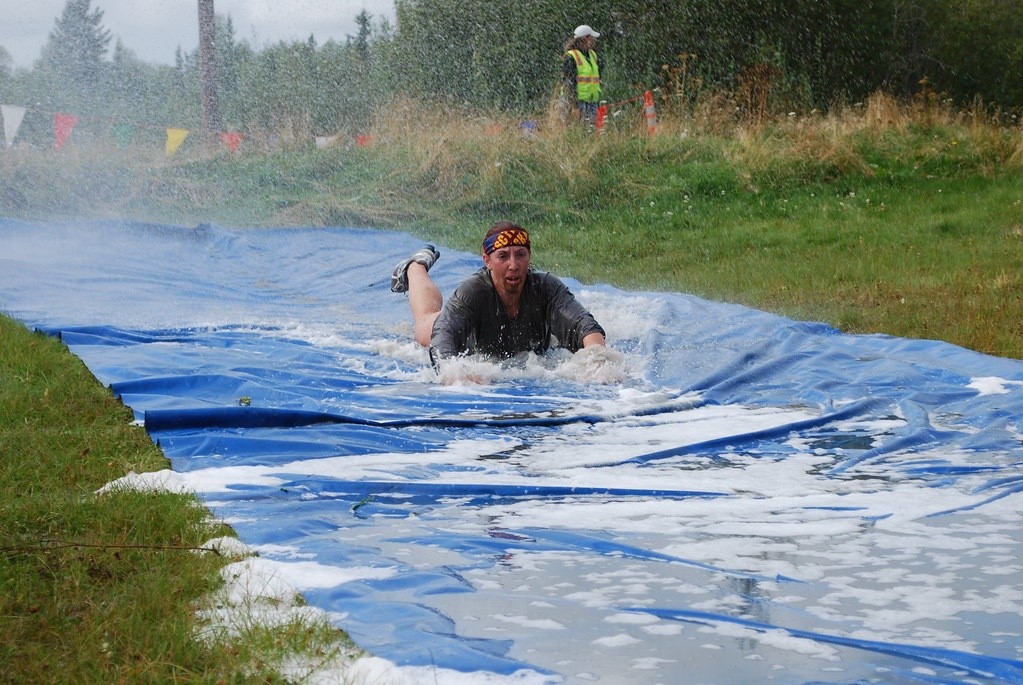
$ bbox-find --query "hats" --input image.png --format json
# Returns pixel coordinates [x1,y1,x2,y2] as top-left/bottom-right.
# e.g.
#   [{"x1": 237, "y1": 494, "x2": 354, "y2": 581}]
[{"x1": 573, "y1": 24, "x2": 600, "y2": 39}]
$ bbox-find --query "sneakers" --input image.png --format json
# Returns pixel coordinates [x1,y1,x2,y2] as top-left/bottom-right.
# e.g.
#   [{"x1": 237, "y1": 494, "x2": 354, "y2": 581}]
[{"x1": 391, "y1": 244, "x2": 440, "y2": 295}]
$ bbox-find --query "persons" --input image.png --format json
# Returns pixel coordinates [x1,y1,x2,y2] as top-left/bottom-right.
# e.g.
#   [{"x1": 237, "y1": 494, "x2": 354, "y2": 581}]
[
  {"x1": 564, "y1": 23, "x2": 603, "y2": 126},
  {"x1": 390, "y1": 220, "x2": 623, "y2": 389}
]
